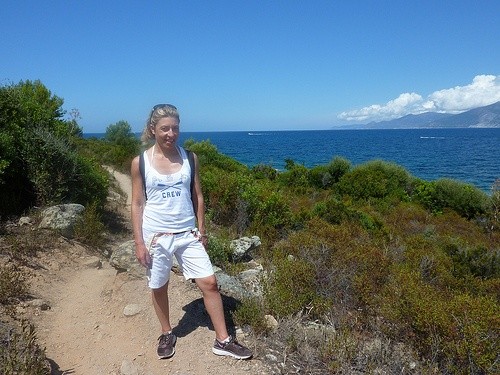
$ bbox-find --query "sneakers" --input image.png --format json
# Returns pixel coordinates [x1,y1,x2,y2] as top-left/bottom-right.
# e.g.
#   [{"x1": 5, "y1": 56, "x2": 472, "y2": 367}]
[
  {"x1": 212, "y1": 334, "x2": 252, "y2": 359},
  {"x1": 157, "y1": 330, "x2": 177, "y2": 359}
]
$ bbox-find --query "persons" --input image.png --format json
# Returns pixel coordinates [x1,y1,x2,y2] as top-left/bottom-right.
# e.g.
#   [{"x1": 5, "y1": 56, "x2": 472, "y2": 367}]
[{"x1": 129, "y1": 102, "x2": 254, "y2": 360}]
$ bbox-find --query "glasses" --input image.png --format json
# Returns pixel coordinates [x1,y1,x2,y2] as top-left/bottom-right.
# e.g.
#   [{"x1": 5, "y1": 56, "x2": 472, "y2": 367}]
[{"x1": 149, "y1": 104, "x2": 177, "y2": 124}]
